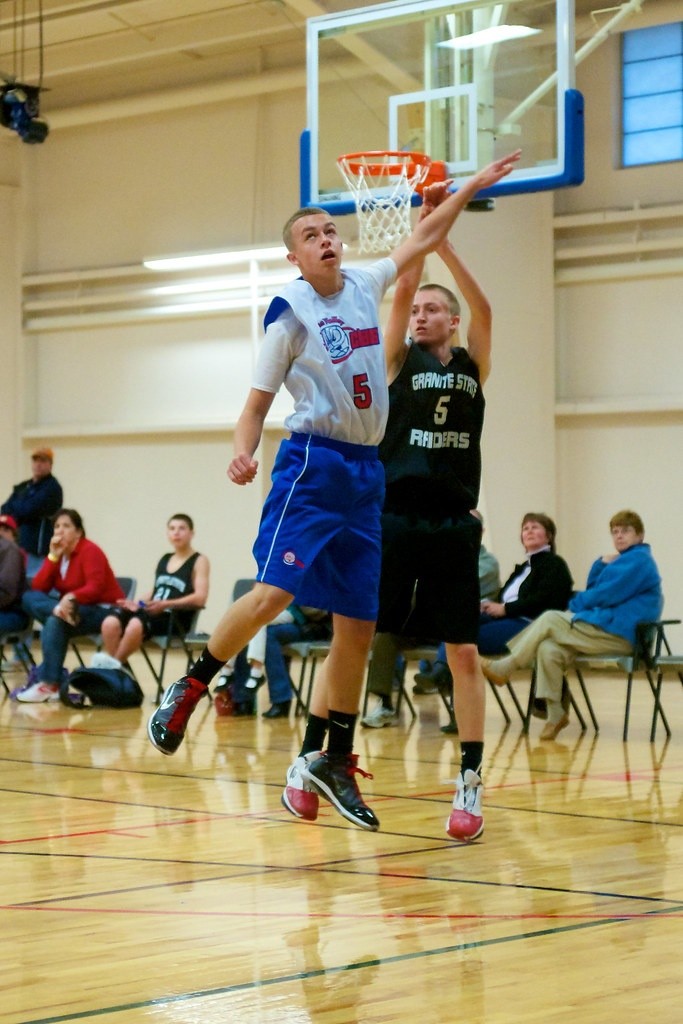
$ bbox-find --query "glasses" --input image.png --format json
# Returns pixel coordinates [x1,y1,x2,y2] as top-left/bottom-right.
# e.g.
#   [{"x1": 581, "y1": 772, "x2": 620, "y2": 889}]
[{"x1": 32, "y1": 454, "x2": 49, "y2": 462}]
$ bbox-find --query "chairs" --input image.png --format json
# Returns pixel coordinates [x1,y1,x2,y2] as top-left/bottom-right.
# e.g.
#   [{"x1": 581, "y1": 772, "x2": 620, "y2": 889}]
[
  {"x1": 138, "y1": 608, "x2": 201, "y2": 707},
  {"x1": 183, "y1": 577, "x2": 683, "y2": 742},
  {"x1": 67, "y1": 575, "x2": 137, "y2": 708},
  {"x1": 0, "y1": 579, "x2": 39, "y2": 696}
]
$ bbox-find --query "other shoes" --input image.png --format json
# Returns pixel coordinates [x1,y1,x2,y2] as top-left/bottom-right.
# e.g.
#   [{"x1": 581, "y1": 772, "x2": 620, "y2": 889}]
[
  {"x1": 414, "y1": 663, "x2": 452, "y2": 690},
  {"x1": 440, "y1": 724, "x2": 458, "y2": 733},
  {"x1": 239, "y1": 675, "x2": 265, "y2": 696},
  {"x1": 213, "y1": 672, "x2": 234, "y2": 692},
  {"x1": 262, "y1": 701, "x2": 291, "y2": 718},
  {"x1": 479, "y1": 655, "x2": 509, "y2": 687},
  {"x1": 539, "y1": 711, "x2": 569, "y2": 740}
]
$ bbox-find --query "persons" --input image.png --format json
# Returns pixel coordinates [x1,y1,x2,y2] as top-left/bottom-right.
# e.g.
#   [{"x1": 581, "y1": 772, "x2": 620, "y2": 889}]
[
  {"x1": 222, "y1": 604, "x2": 340, "y2": 720},
  {"x1": 15, "y1": 508, "x2": 126, "y2": 703},
  {"x1": 360, "y1": 509, "x2": 499, "y2": 738},
  {"x1": 0, "y1": 516, "x2": 27, "y2": 650},
  {"x1": 0, "y1": 449, "x2": 63, "y2": 673},
  {"x1": 148, "y1": 150, "x2": 525, "y2": 833},
  {"x1": 280, "y1": 179, "x2": 499, "y2": 844},
  {"x1": 412, "y1": 512, "x2": 577, "y2": 735},
  {"x1": 479, "y1": 510, "x2": 664, "y2": 740},
  {"x1": 69, "y1": 514, "x2": 210, "y2": 710}
]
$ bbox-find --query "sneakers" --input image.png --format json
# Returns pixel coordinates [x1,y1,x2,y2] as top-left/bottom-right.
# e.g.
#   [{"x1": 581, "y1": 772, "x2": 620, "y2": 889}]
[
  {"x1": 360, "y1": 707, "x2": 399, "y2": 727},
  {"x1": 306, "y1": 751, "x2": 380, "y2": 832},
  {"x1": 16, "y1": 681, "x2": 60, "y2": 703},
  {"x1": 445, "y1": 762, "x2": 485, "y2": 843},
  {"x1": 147, "y1": 676, "x2": 209, "y2": 756},
  {"x1": 280, "y1": 751, "x2": 322, "y2": 821},
  {"x1": 2, "y1": 660, "x2": 32, "y2": 673},
  {"x1": 60, "y1": 598, "x2": 80, "y2": 626}
]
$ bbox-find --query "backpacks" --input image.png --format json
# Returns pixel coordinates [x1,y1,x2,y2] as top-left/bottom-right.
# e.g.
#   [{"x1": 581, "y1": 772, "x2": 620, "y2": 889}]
[{"x1": 60, "y1": 667, "x2": 144, "y2": 709}]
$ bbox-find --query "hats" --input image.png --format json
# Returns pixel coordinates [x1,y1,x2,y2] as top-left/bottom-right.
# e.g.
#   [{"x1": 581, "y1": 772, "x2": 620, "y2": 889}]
[{"x1": 0, "y1": 515, "x2": 17, "y2": 529}]
